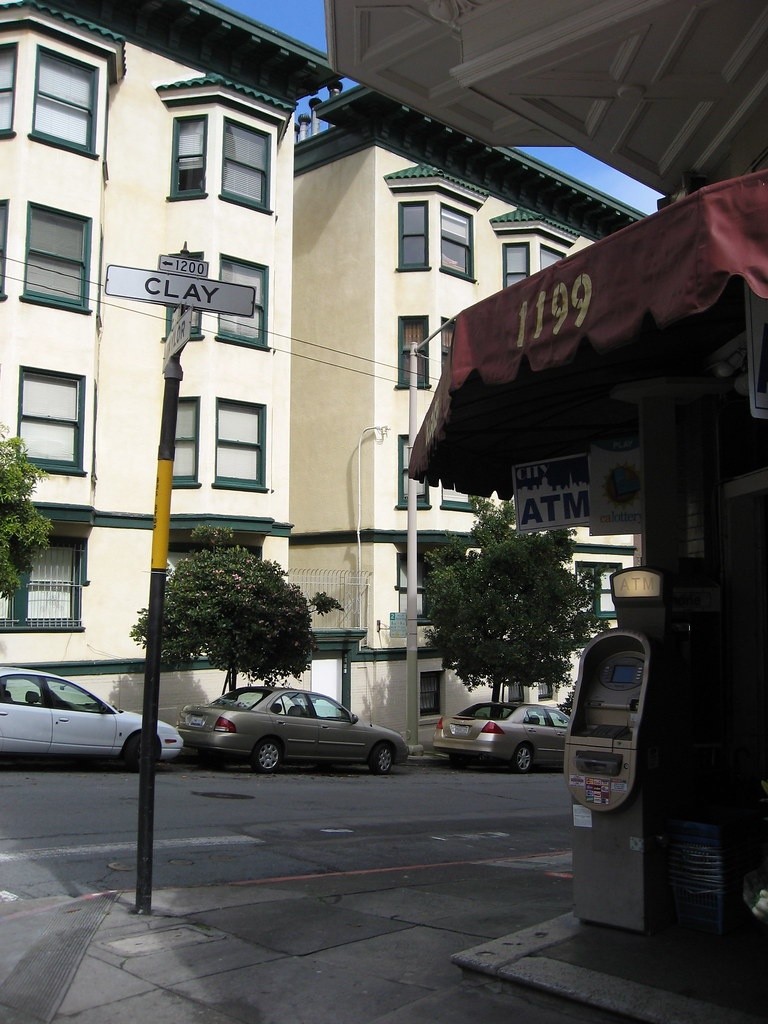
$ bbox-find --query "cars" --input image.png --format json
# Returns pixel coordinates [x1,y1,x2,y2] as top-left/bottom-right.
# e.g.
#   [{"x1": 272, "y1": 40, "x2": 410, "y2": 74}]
[
  {"x1": 177, "y1": 686, "x2": 409, "y2": 774},
  {"x1": 432, "y1": 701, "x2": 569, "y2": 774},
  {"x1": 0, "y1": 666, "x2": 183, "y2": 774}
]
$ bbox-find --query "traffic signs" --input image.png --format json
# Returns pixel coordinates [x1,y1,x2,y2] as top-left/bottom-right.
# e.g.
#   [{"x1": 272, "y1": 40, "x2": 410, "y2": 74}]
[
  {"x1": 162, "y1": 302, "x2": 192, "y2": 375},
  {"x1": 104, "y1": 253, "x2": 259, "y2": 318}
]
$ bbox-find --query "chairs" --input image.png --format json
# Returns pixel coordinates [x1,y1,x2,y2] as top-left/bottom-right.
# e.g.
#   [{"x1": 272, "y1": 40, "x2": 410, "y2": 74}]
[
  {"x1": 528, "y1": 715, "x2": 540, "y2": 725},
  {"x1": 270, "y1": 704, "x2": 282, "y2": 714},
  {"x1": 0, "y1": 683, "x2": 13, "y2": 704},
  {"x1": 288, "y1": 706, "x2": 301, "y2": 716},
  {"x1": 25, "y1": 691, "x2": 39, "y2": 705}
]
